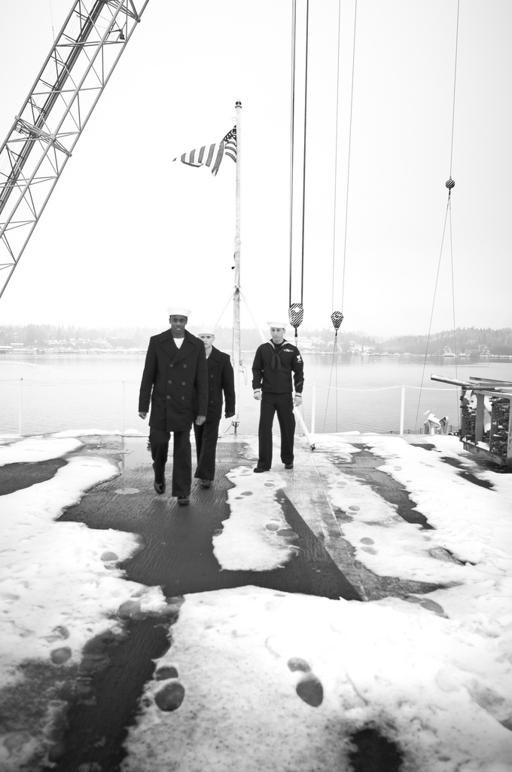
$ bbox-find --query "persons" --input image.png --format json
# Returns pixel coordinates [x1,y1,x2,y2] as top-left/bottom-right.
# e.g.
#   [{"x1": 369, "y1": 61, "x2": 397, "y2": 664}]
[
  {"x1": 251, "y1": 321, "x2": 304, "y2": 472},
  {"x1": 139, "y1": 309, "x2": 235, "y2": 506}
]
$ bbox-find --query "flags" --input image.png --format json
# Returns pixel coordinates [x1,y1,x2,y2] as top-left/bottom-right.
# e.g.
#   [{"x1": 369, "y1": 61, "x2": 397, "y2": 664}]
[{"x1": 172, "y1": 126, "x2": 236, "y2": 176}]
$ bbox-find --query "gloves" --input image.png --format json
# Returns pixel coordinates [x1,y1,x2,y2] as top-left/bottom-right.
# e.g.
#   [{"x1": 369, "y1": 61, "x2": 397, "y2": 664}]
[
  {"x1": 293, "y1": 393, "x2": 303, "y2": 406},
  {"x1": 225, "y1": 412, "x2": 234, "y2": 418},
  {"x1": 253, "y1": 388, "x2": 261, "y2": 400}
]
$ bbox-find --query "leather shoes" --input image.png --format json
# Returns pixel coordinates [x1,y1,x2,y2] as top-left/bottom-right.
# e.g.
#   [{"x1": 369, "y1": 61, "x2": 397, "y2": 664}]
[
  {"x1": 177, "y1": 496, "x2": 190, "y2": 505},
  {"x1": 201, "y1": 478, "x2": 211, "y2": 488},
  {"x1": 285, "y1": 460, "x2": 294, "y2": 469},
  {"x1": 154, "y1": 482, "x2": 166, "y2": 494},
  {"x1": 254, "y1": 465, "x2": 270, "y2": 472}
]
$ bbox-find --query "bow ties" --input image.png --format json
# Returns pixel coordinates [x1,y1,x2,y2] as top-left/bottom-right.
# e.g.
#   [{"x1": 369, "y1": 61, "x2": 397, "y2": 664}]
[{"x1": 270, "y1": 347, "x2": 284, "y2": 370}]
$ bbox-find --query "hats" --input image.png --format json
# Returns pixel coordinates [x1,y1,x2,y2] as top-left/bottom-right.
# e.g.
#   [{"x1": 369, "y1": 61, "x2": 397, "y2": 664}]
[
  {"x1": 196, "y1": 325, "x2": 215, "y2": 336},
  {"x1": 167, "y1": 307, "x2": 192, "y2": 317},
  {"x1": 267, "y1": 318, "x2": 286, "y2": 328}
]
